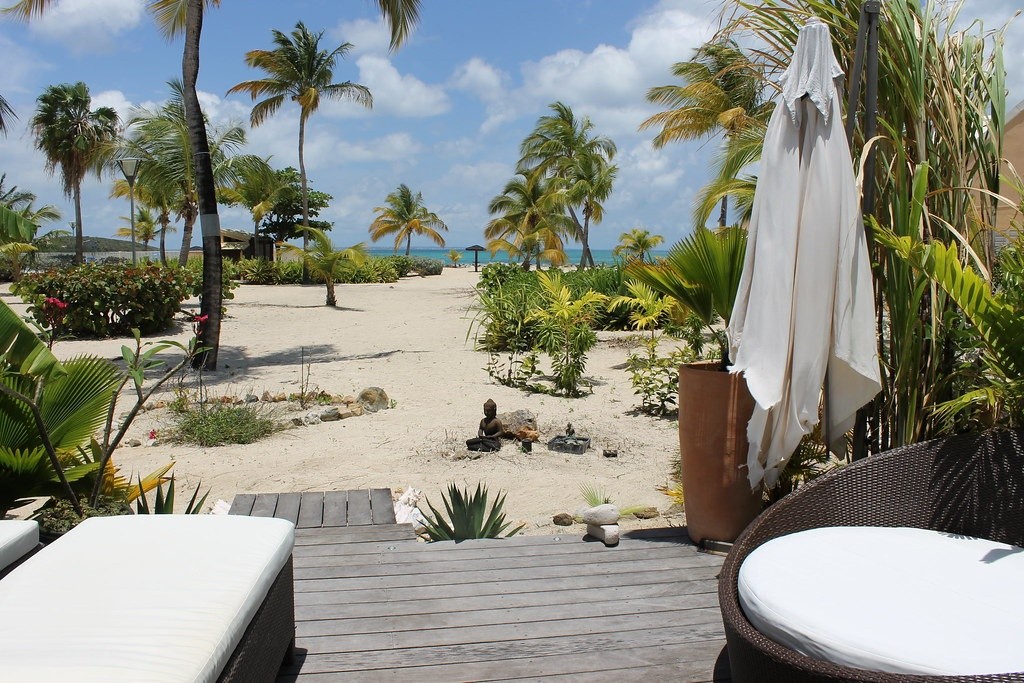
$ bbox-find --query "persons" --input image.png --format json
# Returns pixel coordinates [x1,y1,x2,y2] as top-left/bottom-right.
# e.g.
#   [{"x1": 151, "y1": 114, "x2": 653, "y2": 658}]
[{"x1": 466, "y1": 399, "x2": 503, "y2": 452}]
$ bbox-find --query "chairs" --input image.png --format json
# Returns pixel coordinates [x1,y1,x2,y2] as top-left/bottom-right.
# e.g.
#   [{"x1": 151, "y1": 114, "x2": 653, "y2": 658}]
[{"x1": 719, "y1": 424, "x2": 1024, "y2": 683}]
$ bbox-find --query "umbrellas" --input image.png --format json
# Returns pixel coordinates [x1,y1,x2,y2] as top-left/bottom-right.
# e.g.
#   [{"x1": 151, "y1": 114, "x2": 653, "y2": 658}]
[
  {"x1": 725, "y1": 14, "x2": 884, "y2": 489},
  {"x1": 466, "y1": 245, "x2": 487, "y2": 271}
]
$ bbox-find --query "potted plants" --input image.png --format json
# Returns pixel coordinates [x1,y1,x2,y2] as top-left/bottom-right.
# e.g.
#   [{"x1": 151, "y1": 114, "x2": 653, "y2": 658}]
[
  {"x1": 621, "y1": 223, "x2": 765, "y2": 544},
  {"x1": 547, "y1": 421, "x2": 591, "y2": 454}
]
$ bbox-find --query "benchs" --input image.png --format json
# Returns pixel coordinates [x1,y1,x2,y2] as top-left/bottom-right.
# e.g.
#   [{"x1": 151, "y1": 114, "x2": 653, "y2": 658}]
[{"x1": 0, "y1": 513, "x2": 301, "y2": 683}]
